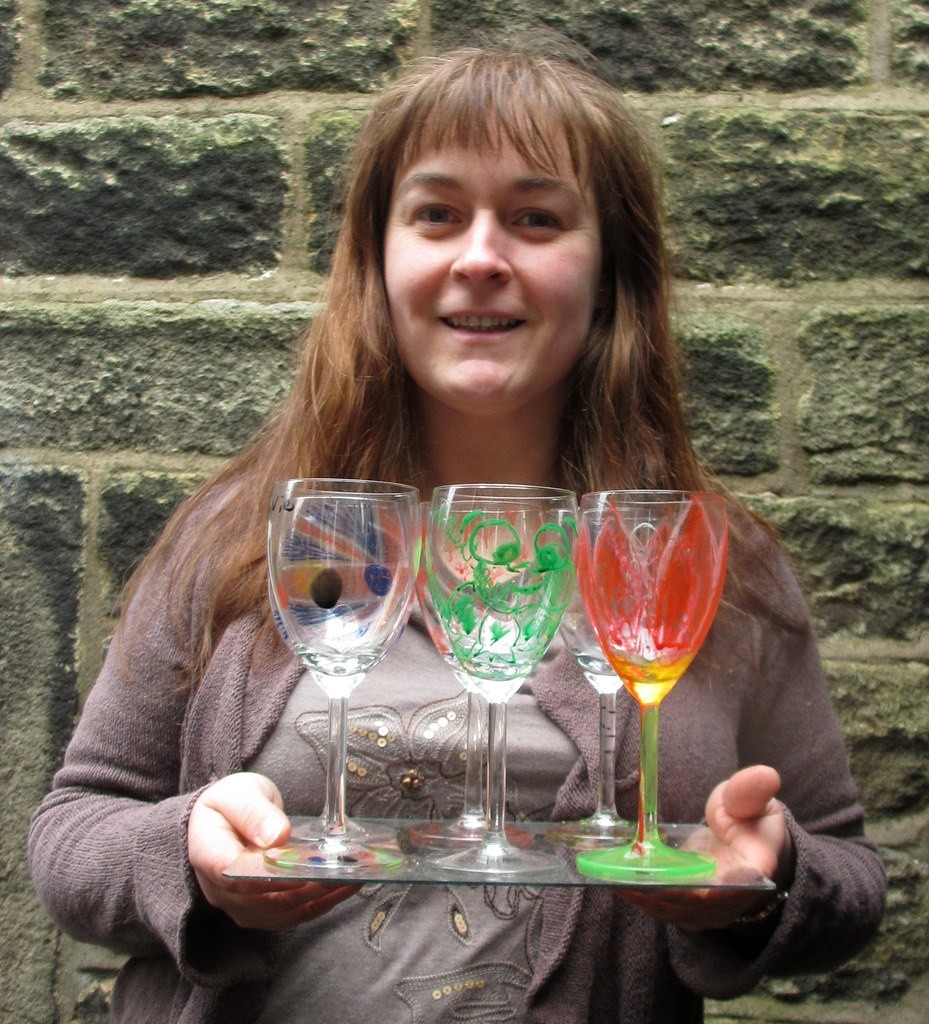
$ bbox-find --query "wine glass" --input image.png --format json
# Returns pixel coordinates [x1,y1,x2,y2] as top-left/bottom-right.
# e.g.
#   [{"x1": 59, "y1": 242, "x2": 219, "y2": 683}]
[{"x1": 261, "y1": 476, "x2": 729, "y2": 877}]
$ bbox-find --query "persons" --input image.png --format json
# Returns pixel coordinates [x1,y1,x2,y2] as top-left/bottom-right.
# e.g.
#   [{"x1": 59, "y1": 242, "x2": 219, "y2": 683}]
[{"x1": 24, "y1": 46, "x2": 885, "y2": 1022}]
